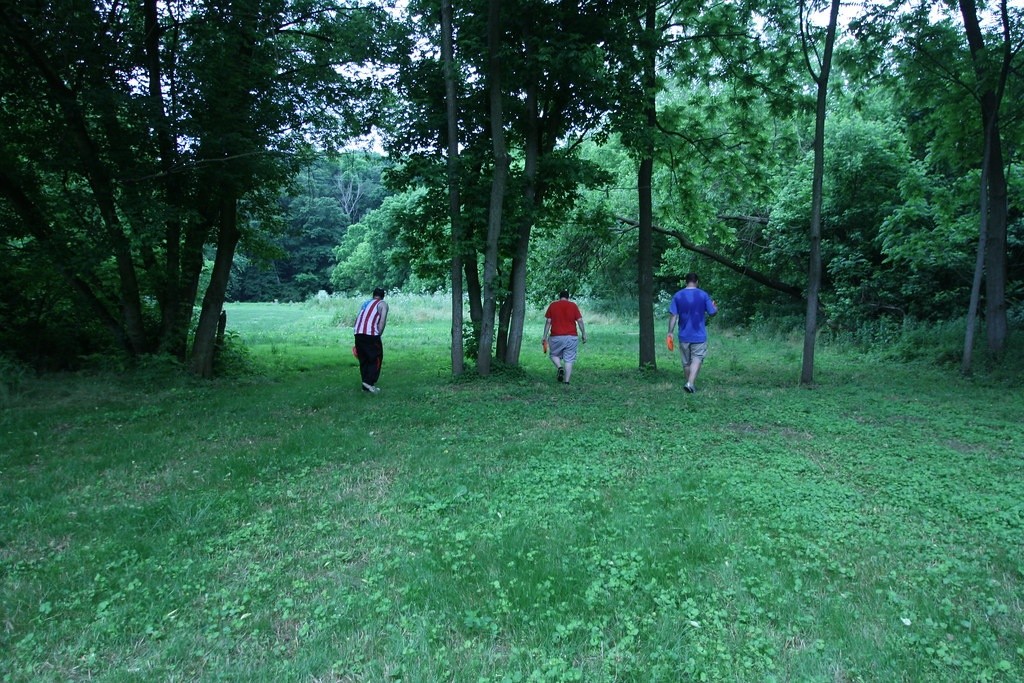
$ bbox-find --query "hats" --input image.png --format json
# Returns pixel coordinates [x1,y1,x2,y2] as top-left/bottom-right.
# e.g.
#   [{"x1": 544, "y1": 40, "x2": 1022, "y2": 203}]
[
  {"x1": 373, "y1": 288, "x2": 384, "y2": 298},
  {"x1": 560, "y1": 291, "x2": 569, "y2": 299},
  {"x1": 685, "y1": 273, "x2": 699, "y2": 284}
]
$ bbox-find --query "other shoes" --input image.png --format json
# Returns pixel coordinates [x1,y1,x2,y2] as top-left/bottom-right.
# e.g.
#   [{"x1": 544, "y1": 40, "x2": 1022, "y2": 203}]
[
  {"x1": 684, "y1": 382, "x2": 696, "y2": 394},
  {"x1": 364, "y1": 382, "x2": 376, "y2": 393},
  {"x1": 557, "y1": 367, "x2": 564, "y2": 383},
  {"x1": 361, "y1": 387, "x2": 380, "y2": 392},
  {"x1": 564, "y1": 382, "x2": 570, "y2": 386}
]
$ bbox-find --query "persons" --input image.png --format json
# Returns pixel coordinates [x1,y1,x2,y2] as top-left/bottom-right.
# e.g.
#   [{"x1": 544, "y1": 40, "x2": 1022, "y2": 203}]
[
  {"x1": 541, "y1": 291, "x2": 587, "y2": 385},
  {"x1": 353, "y1": 287, "x2": 389, "y2": 394},
  {"x1": 666, "y1": 271, "x2": 718, "y2": 394}
]
